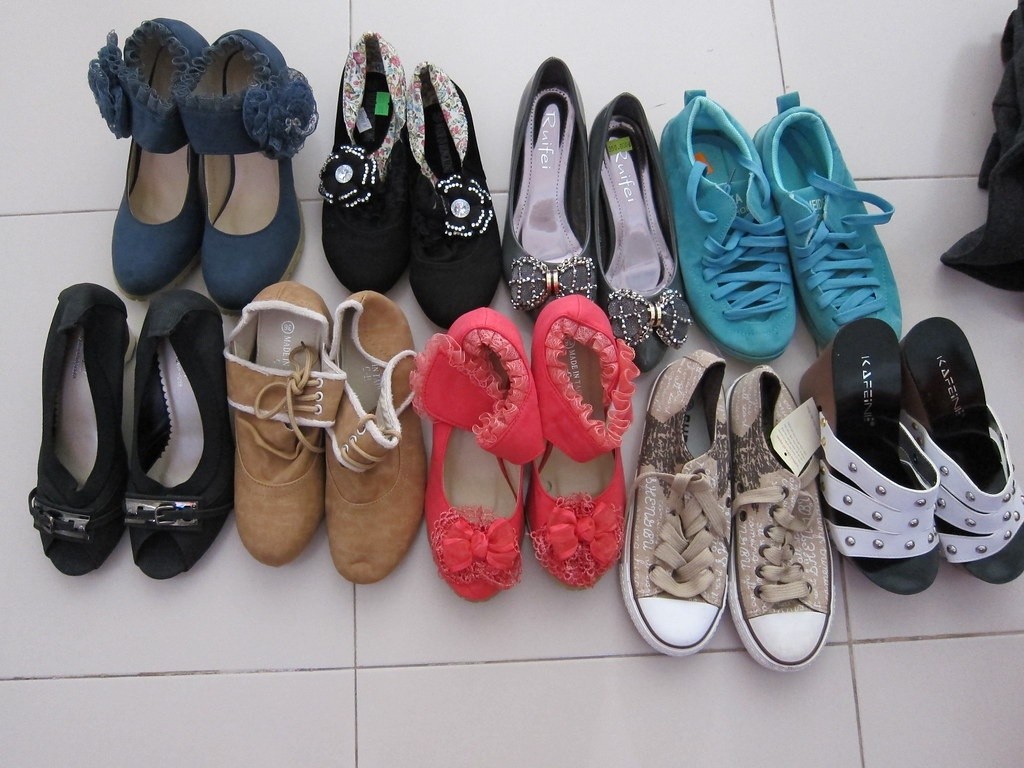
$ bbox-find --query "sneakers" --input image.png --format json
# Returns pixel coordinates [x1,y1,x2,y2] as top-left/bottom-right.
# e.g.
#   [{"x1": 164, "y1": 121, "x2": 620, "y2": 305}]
[
  {"x1": 617, "y1": 349, "x2": 732, "y2": 658},
  {"x1": 749, "y1": 91, "x2": 902, "y2": 346},
  {"x1": 727, "y1": 364, "x2": 835, "y2": 673},
  {"x1": 659, "y1": 90, "x2": 797, "y2": 363}
]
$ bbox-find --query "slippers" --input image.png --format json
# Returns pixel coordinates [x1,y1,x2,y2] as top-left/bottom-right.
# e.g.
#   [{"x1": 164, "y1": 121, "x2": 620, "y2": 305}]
[
  {"x1": 820, "y1": 318, "x2": 940, "y2": 594},
  {"x1": 898, "y1": 316, "x2": 1024, "y2": 584}
]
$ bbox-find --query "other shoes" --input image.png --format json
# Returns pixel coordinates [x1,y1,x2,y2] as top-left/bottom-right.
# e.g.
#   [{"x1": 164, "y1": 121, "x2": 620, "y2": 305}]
[
  {"x1": 122, "y1": 287, "x2": 236, "y2": 580},
  {"x1": 402, "y1": 62, "x2": 502, "y2": 328},
  {"x1": 525, "y1": 294, "x2": 639, "y2": 592},
  {"x1": 410, "y1": 307, "x2": 545, "y2": 603},
  {"x1": 28, "y1": 283, "x2": 137, "y2": 577},
  {"x1": 323, "y1": 291, "x2": 426, "y2": 585},
  {"x1": 318, "y1": 33, "x2": 407, "y2": 294},
  {"x1": 501, "y1": 57, "x2": 597, "y2": 324},
  {"x1": 174, "y1": 29, "x2": 322, "y2": 317},
  {"x1": 87, "y1": 19, "x2": 210, "y2": 302},
  {"x1": 222, "y1": 280, "x2": 334, "y2": 566},
  {"x1": 591, "y1": 92, "x2": 692, "y2": 372}
]
